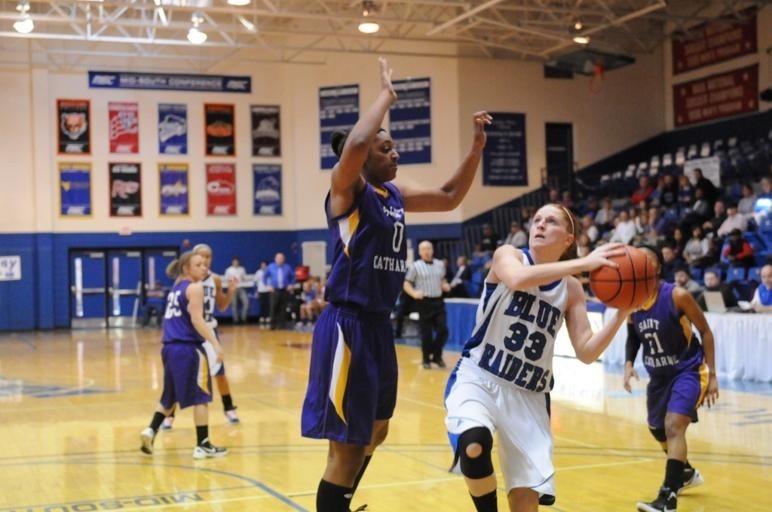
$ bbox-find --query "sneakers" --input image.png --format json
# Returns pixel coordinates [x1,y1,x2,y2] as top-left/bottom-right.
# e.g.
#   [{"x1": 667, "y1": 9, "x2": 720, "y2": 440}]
[
  {"x1": 193, "y1": 442, "x2": 228, "y2": 460},
  {"x1": 224, "y1": 410, "x2": 238, "y2": 423},
  {"x1": 140, "y1": 427, "x2": 155, "y2": 454},
  {"x1": 637, "y1": 488, "x2": 676, "y2": 512},
  {"x1": 676, "y1": 468, "x2": 704, "y2": 495},
  {"x1": 160, "y1": 417, "x2": 173, "y2": 431}
]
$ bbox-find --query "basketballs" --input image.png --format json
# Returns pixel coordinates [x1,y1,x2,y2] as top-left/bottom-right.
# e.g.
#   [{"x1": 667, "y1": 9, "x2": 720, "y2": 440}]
[{"x1": 589, "y1": 246, "x2": 657, "y2": 309}]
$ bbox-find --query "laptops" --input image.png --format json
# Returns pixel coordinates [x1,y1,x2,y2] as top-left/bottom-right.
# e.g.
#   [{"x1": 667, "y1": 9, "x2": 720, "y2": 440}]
[{"x1": 703, "y1": 290, "x2": 739, "y2": 312}]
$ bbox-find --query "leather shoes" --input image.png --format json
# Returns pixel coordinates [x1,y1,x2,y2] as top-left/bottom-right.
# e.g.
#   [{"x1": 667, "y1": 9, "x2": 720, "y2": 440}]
[
  {"x1": 423, "y1": 362, "x2": 430, "y2": 369},
  {"x1": 434, "y1": 359, "x2": 446, "y2": 367}
]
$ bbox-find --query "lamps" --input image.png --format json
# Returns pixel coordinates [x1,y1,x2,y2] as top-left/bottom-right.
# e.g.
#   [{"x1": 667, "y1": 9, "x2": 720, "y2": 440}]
[{"x1": 357, "y1": 0, "x2": 382, "y2": 35}]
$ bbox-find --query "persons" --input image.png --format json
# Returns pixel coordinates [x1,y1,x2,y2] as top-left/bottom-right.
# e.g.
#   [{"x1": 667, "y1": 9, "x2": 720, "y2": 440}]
[{"x1": 302, "y1": 56, "x2": 494, "y2": 512}]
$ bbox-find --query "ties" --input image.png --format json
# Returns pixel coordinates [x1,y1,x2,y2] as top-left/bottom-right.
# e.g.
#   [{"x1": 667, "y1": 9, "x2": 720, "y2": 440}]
[{"x1": 277, "y1": 267, "x2": 284, "y2": 290}]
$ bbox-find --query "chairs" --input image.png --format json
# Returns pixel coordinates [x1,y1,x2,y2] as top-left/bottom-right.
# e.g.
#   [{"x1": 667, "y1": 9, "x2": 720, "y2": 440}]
[
  {"x1": 562, "y1": 125, "x2": 771, "y2": 312},
  {"x1": 445, "y1": 211, "x2": 538, "y2": 302}
]
